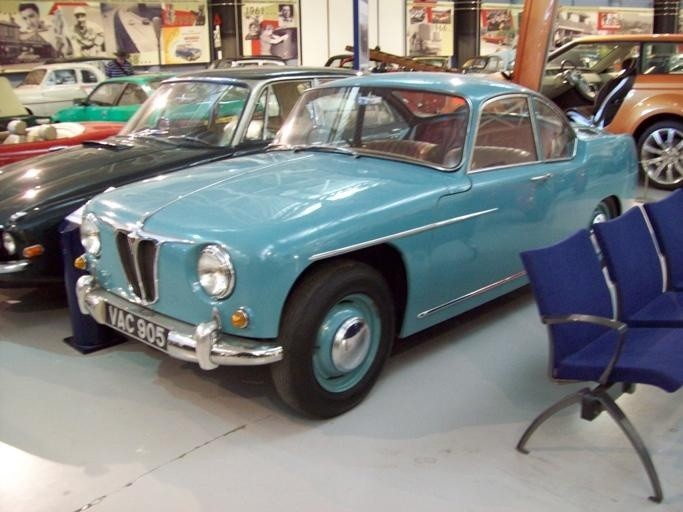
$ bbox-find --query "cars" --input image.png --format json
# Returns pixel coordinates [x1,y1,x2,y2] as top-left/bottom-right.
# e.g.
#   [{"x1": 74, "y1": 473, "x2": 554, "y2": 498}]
[
  {"x1": 410, "y1": 6, "x2": 425, "y2": 24},
  {"x1": 431, "y1": 9, "x2": 450, "y2": 23}
]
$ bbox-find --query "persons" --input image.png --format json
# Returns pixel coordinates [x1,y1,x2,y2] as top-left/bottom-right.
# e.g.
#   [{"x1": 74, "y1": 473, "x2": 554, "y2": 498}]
[
  {"x1": 105, "y1": 49, "x2": 135, "y2": 78},
  {"x1": 259, "y1": 25, "x2": 289, "y2": 55},
  {"x1": 17, "y1": 3, "x2": 69, "y2": 50},
  {"x1": 278, "y1": 5, "x2": 293, "y2": 23},
  {"x1": 70, "y1": 6, "x2": 105, "y2": 55},
  {"x1": 246, "y1": 23, "x2": 261, "y2": 40}
]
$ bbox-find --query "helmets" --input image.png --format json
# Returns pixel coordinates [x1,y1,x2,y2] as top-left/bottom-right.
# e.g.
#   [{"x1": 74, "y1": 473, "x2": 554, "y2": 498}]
[{"x1": 72, "y1": 6, "x2": 86, "y2": 16}]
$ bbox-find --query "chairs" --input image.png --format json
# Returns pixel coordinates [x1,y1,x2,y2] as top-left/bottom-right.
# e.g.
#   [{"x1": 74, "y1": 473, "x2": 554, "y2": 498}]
[
  {"x1": 592, "y1": 206, "x2": 681, "y2": 328},
  {"x1": 516, "y1": 234, "x2": 682, "y2": 505},
  {"x1": 367, "y1": 138, "x2": 437, "y2": 162},
  {"x1": 442, "y1": 144, "x2": 533, "y2": 175},
  {"x1": 644, "y1": 189, "x2": 682, "y2": 289}
]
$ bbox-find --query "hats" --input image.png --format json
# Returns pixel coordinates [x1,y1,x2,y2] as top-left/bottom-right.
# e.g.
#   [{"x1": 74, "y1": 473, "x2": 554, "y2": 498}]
[{"x1": 111, "y1": 50, "x2": 130, "y2": 59}]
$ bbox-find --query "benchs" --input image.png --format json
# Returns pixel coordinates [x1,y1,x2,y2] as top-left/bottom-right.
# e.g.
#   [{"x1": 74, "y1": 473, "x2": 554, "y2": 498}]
[{"x1": 411, "y1": 114, "x2": 559, "y2": 160}]
